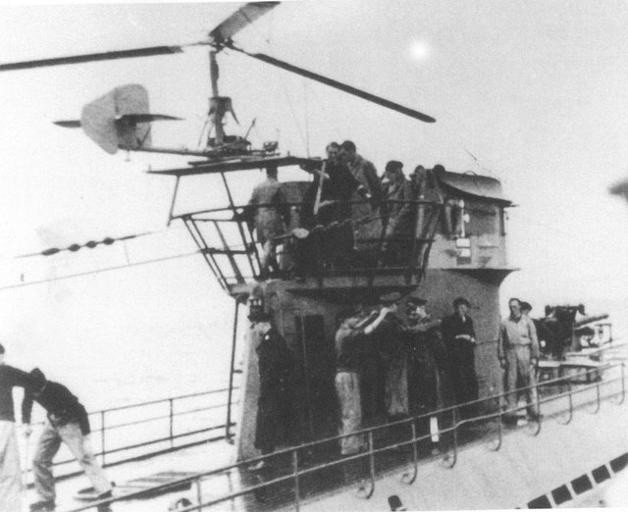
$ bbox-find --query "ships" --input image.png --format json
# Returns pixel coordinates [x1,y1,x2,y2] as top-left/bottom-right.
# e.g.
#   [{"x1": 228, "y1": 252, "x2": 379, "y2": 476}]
[{"x1": 0, "y1": 171, "x2": 628, "y2": 512}]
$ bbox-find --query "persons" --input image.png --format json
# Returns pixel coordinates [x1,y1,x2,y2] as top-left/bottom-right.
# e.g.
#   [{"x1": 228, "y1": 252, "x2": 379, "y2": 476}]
[
  {"x1": 247, "y1": 313, "x2": 290, "y2": 471},
  {"x1": 0, "y1": 344, "x2": 43, "y2": 511},
  {"x1": 22, "y1": 369, "x2": 111, "y2": 510},
  {"x1": 335, "y1": 299, "x2": 540, "y2": 461},
  {"x1": 245, "y1": 141, "x2": 414, "y2": 278}
]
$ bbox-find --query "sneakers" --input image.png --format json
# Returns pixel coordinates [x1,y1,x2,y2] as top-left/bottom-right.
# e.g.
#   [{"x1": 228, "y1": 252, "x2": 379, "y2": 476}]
[{"x1": 31, "y1": 499, "x2": 55, "y2": 511}]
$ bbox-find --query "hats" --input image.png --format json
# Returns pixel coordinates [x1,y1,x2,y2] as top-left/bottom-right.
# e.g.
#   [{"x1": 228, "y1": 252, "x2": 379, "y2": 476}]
[
  {"x1": 386, "y1": 160, "x2": 402, "y2": 174},
  {"x1": 405, "y1": 296, "x2": 428, "y2": 306},
  {"x1": 453, "y1": 297, "x2": 471, "y2": 308},
  {"x1": 380, "y1": 291, "x2": 402, "y2": 306},
  {"x1": 338, "y1": 304, "x2": 363, "y2": 321}
]
